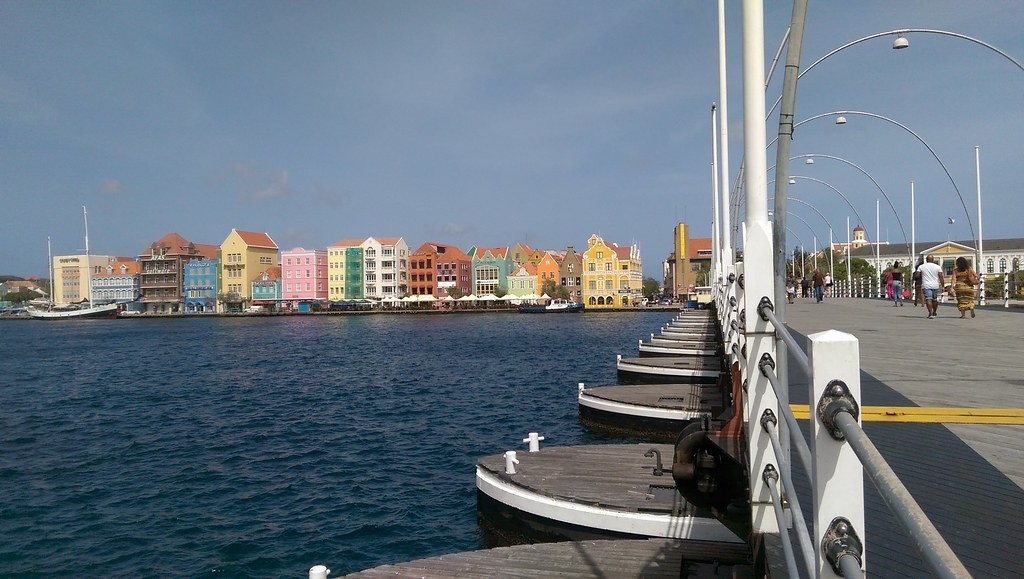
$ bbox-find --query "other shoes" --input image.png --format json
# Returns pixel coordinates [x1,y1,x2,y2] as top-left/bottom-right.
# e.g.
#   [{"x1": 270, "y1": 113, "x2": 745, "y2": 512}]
[
  {"x1": 933, "y1": 312, "x2": 937, "y2": 316},
  {"x1": 960, "y1": 315, "x2": 965, "y2": 318},
  {"x1": 929, "y1": 315, "x2": 934, "y2": 318},
  {"x1": 971, "y1": 308, "x2": 975, "y2": 318}
]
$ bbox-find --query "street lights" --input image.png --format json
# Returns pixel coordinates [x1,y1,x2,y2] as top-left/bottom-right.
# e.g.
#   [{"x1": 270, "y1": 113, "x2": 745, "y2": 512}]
[
  {"x1": 624, "y1": 286, "x2": 631, "y2": 299},
  {"x1": 641, "y1": 286, "x2": 645, "y2": 297}
]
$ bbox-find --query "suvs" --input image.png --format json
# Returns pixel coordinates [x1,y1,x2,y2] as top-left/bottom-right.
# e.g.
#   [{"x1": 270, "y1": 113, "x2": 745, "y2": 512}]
[
  {"x1": 225, "y1": 308, "x2": 242, "y2": 313},
  {"x1": 117, "y1": 308, "x2": 125, "y2": 314},
  {"x1": 672, "y1": 299, "x2": 683, "y2": 305}
]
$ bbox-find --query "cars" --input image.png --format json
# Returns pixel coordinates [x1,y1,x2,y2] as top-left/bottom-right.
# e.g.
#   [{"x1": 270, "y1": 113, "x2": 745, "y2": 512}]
[
  {"x1": 646, "y1": 300, "x2": 661, "y2": 307},
  {"x1": 661, "y1": 298, "x2": 672, "y2": 305},
  {"x1": 121, "y1": 310, "x2": 140, "y2": 315}
]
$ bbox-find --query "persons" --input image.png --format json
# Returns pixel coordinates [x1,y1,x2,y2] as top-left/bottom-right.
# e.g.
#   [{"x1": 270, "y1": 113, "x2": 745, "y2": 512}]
[
  {"x1": 891, "y1": 254, "x2": 976, "y2": 319},
  {"x1": 785, "y1": 272, "x2": 832, "y2": 304}
]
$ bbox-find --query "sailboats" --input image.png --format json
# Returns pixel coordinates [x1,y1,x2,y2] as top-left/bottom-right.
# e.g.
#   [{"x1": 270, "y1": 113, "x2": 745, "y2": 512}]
[{"x1": 25, "y1": 205, "x2": 120, "y2": 319}]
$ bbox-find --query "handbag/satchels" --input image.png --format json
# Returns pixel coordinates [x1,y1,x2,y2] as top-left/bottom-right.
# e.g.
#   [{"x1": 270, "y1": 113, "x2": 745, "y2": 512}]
[
  {"x1": 902, "y1": 288, "x2": 911, "y2": 298},
  {"x1": 883, "y1": 277, "x2": 888, "y2": 284},
  {"x1": 968, "y1": 267, "x2": 979, "y2": 284}
]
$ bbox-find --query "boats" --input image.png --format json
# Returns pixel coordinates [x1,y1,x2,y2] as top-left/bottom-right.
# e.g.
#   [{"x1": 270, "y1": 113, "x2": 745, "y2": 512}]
[{"x1": 519, "y1": 298, "x2": 584, "y2": 315}]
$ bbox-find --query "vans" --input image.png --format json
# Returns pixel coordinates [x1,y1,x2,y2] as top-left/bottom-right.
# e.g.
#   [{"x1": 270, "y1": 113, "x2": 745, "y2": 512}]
[{"x1": 244, "y1": 306, "x2": 263, "y2": 313}]
